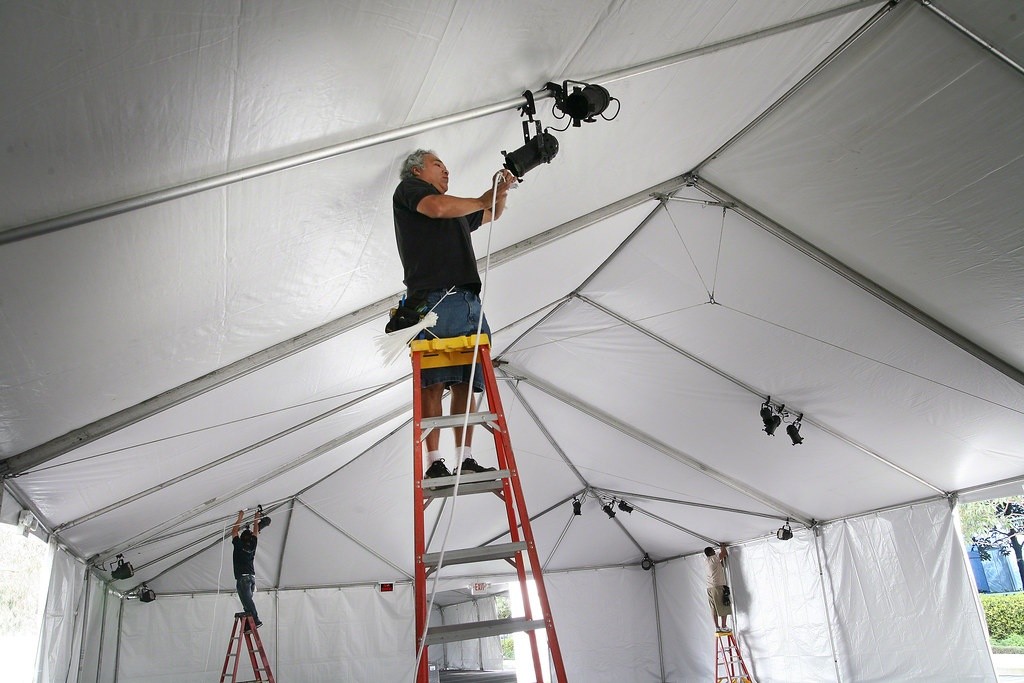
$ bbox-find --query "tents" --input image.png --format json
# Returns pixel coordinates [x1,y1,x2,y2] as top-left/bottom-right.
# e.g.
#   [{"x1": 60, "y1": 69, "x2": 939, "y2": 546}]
[{"x1": 968, "y1": 534, "x2": 1024, "y2": 594}]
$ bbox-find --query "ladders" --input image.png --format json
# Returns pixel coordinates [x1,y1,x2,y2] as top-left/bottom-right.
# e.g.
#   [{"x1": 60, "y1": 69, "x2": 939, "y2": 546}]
[
  {"x1": 220, "y1": 612, "x2": 276, "y2": 683},
  {"x1": 409, "y1": 333, "x2": 569, "y2": 683},
  {"x1": 715, "y1": 632, "x2": 752, "y2": 683}
]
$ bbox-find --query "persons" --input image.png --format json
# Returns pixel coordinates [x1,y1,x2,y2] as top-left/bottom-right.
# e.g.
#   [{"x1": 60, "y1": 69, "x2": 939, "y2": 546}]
[
  {"x1": 704, "y1": 543, "x2": 732, "y2": 632},
  {"x1": 393, "y1": 149, "x2": 516, "y2": 490},
  {"x1": 232, "y1": 510, "x2": 263, "y2": 635}
]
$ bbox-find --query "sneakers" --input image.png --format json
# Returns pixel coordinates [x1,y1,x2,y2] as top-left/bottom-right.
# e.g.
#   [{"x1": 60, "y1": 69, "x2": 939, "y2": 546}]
[
  {"x1": 424, "y1": 458, "x2": 456, "y2": 491},
  {"x1": 453, "y1": 458, "x2": 497, "y2": 474}
]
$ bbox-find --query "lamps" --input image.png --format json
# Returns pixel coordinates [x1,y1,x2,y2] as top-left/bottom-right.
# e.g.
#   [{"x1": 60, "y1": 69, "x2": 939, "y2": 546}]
[
  {"x1": 253, "y1": 507, "x2": 271, "y2": 530},
  {"x1": 787, "y1": 413, "x2": 804, "y2": 445},
  {"x1": 549, "y1": 79, "x2": 622, "y2": 133},
  {"x1": 642, "y1": 553, "x2": 653, "y2": 571},
  {"x1": 573, "y1": 496, "x2": 581, "y2": 517},
  {"x1": 601, "y1": 501, "x2": 617, "y2": 518},
  {"x1": 760, "y1": 396, "x2": 785, "y2": 436},
  {"x1": 619, "y1": 499, "x2": 634, "y2": 513},
  {"x1": 502, "y1": 90, "x2": 558, "y2": 182},
  {"x1": 777, "y1": 517, "x2": 793, "y2": 540},
  {"x1": 108, "y1": 553, "x2": 135, "y2": 580},
  {"x1": 139, "y1": 583, "x2": 157, "y2": 602}
]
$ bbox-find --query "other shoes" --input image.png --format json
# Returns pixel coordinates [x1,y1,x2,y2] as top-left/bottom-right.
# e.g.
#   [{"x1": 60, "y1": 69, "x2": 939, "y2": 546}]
[
  {"x1": 715, "y1": 627, "x2": 721, "y2": 633},
  {"x1": 720, "y1": 626, "x2": 733, "y2": 632},
  {"x1": 244, "y1": 621, "x2": 263, "y2": 634}
]
